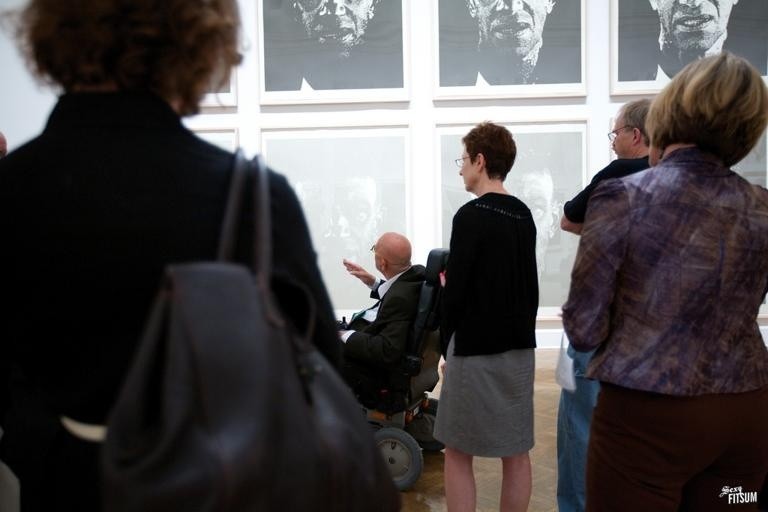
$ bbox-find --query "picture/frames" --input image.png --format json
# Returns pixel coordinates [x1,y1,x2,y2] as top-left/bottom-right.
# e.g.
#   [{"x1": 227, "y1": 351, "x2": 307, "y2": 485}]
[{"x1": 179, "y1": 0, "x2": 767, "y2": 324}]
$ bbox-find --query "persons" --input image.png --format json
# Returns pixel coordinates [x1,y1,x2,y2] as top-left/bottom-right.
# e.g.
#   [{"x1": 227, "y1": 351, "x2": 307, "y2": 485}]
[
  {"x1": 440, "y1": 0, "x2": 579, "y2": 86},
  {"x1": 0, "y1": 0, "x2": 344, "y2": 512},
  {"x1": 336, "y1": 232, "x2": 426, "y2": 407},
  {"x1": 559, "y1": 53, "x2": 768, "y2": 512},
  {"x1": 619, "y1": 0, "x2": 768, "y2": 80},
  {"x1": 428, "y1": 122, "x2": 539, "y2": 512},
  {"x1": 268, "y1": 0, "x2": 397, "y2": 90}
]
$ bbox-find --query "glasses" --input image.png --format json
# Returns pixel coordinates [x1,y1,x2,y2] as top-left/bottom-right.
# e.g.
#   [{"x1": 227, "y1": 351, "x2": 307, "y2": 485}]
[
  {"x1": 455, "y1": 156, "x2": 471, "y2": 168},
  {"x1": 370, "y1": 245, "x2": 383, "y2": 258},
  {"x1": 608, "y1": 127, "x2": 625, "y2": 141}
]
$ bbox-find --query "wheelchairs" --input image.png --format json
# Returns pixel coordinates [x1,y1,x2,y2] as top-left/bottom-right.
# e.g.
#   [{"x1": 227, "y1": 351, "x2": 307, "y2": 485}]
[{"x1": 329, "y1": 246, "x2": 446, "y2": 491}]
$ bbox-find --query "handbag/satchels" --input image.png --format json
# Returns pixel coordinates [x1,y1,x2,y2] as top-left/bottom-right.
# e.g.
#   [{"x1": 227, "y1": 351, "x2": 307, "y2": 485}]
[{"x1": 96, "y1": 148, "x2": 402, "y2": 511}]
